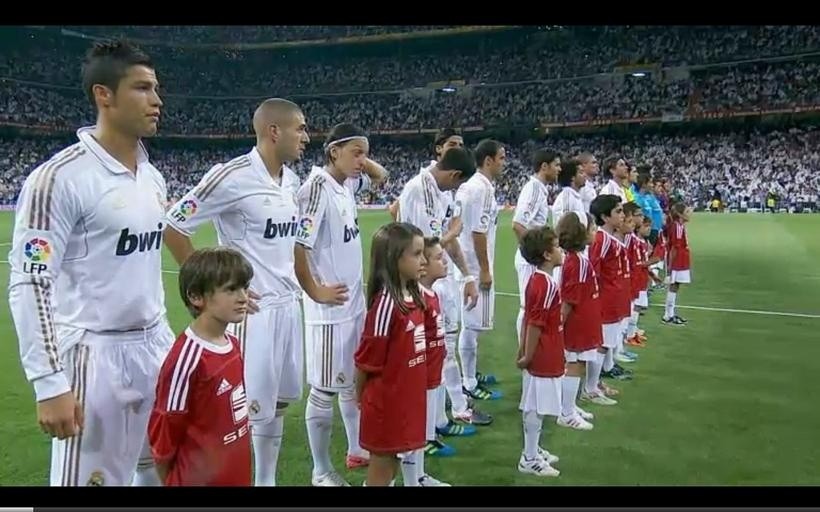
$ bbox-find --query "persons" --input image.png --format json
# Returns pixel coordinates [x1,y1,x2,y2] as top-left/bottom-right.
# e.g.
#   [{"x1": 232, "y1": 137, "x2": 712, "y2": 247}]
[
  {"x1": 294, "y1": 121, "x2": 392, "y2": 486},
  {"x1": 353, "y1": 221, "x2": 428, "y2": 487},
  {"x1": 388, "y1": 126, "x2": 692, "y2": 478},
  {"x1": 1, "y1": 24, "x2": 816, "y2": 213},
  {"x1": 163, "y1": 96, "x2": 311, "y2": 488},
  {"x1": 7, "y1": 37, "x2": 178, "y2": 487},
  {"x1": 147, "y1": 248, "x2": 253, "y2": 487}
]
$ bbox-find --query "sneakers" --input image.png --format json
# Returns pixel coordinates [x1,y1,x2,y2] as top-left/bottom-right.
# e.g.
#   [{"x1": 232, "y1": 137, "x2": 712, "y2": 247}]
[
  {"x1": 532, "y1": 449, "x2": 558, "y2": 465},
  {"x1": 516, "y1": 453, "x2": 560, "y2": 478},
  {"x1": 662, "y1": 315, "x2": 687, "y2": 326},
  {"x1": 599, "y1": 326, "x2": 647, "y2": 379},
  {"x1": 556, "y1": 379, "x2": 619, "y2": 428},
  {"x1": 311, "y1": 372, "x2": 502, "y2": 487}
]
